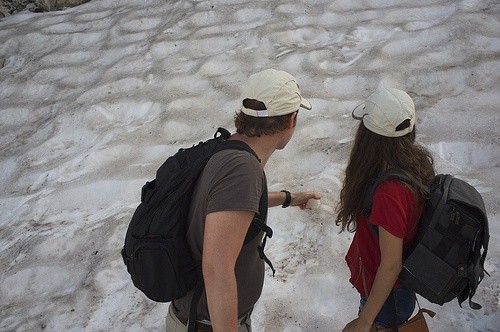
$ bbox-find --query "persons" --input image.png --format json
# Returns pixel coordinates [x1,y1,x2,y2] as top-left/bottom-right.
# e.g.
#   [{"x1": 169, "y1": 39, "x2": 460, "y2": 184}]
[
  {"x1": 336, "y1": 88, "x2": 435, "y2": 332},
  {"x1": 165, "y1": 68, "x2": 321, "y2": 332}
]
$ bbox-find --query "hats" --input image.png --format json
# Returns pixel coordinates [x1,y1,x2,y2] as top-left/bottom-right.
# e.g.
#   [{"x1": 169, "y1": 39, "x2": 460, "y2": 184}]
[
  {"x1": 352, "y1": 88, "x2": 417, "y2": 138},
  {"x1": 241, "y1": 69, "x2": 312, "y2": 118}
]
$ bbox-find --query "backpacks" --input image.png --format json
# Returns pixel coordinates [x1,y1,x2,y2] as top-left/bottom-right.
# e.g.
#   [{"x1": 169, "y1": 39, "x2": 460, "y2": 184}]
[
  {"x1": 121, "y1": 127, "x2": 269, "y2": 303},
  {"x1": 363, "y1": 168, "x2": 491, "y2": 306}
]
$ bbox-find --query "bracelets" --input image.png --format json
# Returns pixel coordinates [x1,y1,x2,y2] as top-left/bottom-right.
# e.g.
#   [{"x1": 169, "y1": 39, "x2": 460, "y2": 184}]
[{"x1": 281, "y1": 190, "x2": 291, "y2": 208}]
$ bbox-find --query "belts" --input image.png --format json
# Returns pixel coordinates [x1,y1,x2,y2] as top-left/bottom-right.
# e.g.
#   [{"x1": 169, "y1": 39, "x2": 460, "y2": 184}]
[{"x1": 170, "y1": 302, "x2": 255, "y2": 332}]
